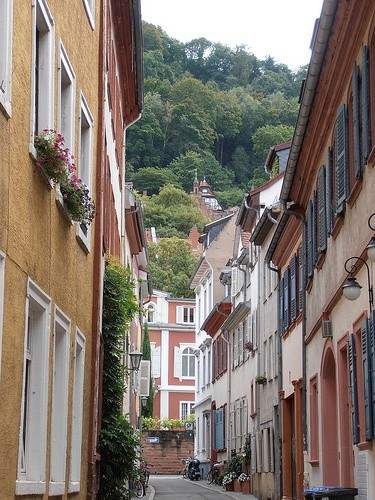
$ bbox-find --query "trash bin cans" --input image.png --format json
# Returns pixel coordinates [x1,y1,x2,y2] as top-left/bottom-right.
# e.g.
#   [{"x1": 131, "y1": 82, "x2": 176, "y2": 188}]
[
  {"x1": 199, "y1": 462, "x2": 211, "y2": 479},
  {"x1": 316, "y1": 488, "x2": 358, "y2": 500},
  {"x1": 304, "y1": 486, "x2": 335, "y2": 500}
]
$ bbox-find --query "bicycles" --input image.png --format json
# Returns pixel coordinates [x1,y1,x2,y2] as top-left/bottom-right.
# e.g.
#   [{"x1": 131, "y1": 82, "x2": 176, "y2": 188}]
[
  {"x1": 132, "y1": 462, "x2": 153, "y2": 498},
  {"x1": 206, "y1": 457, "x2": 222, "y2": 485}
]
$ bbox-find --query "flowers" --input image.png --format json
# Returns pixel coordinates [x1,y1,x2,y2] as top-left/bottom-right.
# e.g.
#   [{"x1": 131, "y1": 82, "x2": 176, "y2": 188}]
[
  {"x1": 222, "y1": 471, "x2": 237, "y2": 486},
  {"x1": 237, "y1": 472, "x2": 250, "y2": 484},
  {"x1": 33, "y1": 128, "x2": 96, "y2": 226}
]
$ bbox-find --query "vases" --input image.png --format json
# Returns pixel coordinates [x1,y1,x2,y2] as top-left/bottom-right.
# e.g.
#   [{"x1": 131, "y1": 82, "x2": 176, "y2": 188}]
[{"x1": 226, "y1": 481, "x2": 251, "y2": 493}]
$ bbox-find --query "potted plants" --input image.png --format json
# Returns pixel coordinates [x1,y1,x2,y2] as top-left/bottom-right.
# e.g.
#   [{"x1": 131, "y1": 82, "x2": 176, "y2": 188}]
[{"x1": 255, "y1": 375, "x2": 266, "y2": 384}]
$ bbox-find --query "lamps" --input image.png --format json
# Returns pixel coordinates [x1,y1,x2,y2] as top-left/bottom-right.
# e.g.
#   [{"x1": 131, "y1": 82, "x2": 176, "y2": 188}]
[
  {"x1": 342, "y1": 256, "x2": 373, "y2": 314},
  {"x1": 366, "y1": 212, "x2": 375, "y2": 262},
  {"x1": 126, "y1": 347, "x2": 144, "y2": 375}
]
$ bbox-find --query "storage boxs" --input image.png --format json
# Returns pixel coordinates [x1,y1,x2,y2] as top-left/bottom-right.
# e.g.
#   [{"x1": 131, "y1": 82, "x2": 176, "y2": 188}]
[{"x1": 191, "y1": 459, "x2": 200, "y2": 467}]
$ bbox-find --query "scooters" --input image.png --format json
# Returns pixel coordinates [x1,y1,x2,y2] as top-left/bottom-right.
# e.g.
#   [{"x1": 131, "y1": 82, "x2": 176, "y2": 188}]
[{"x1": 181, "y1": 455, "x2": 201, "y2": 481}]
